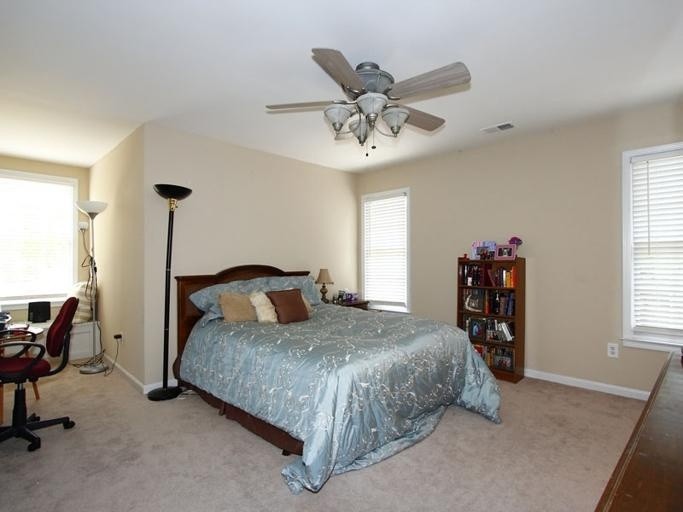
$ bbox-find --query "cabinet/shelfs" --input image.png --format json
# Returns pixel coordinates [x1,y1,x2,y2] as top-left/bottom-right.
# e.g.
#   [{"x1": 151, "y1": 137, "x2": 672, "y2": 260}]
[{"x1": 457, "y1": 256, "x2": 526, "y2": 384}]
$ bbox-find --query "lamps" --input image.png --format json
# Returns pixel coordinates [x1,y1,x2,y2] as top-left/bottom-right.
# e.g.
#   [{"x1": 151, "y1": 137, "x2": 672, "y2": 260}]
[
  {"x1": 148, "y1": 184, "x2": 193, "y2": 401},
  {"x1": 72, "y1": 200, "x2": 108, "y2": 374},
  {"x1": 323, "y1": 86, "x2": 410, "y2": 147},
  {"x1": 315, "y1": 269, "x2": 334, "y2": 304}
]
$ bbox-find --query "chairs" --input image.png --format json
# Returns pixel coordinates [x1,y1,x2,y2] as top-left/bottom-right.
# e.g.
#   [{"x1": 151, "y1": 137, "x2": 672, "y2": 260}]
[{"x1": 0, "y1": 297, "x2": 79, "y2": 452}]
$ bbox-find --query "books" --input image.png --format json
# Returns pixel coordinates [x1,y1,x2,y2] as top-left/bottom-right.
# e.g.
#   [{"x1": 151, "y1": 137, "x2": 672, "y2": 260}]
[{"x1": 463, "y1": 265, "x2": 515, "y2": 369}]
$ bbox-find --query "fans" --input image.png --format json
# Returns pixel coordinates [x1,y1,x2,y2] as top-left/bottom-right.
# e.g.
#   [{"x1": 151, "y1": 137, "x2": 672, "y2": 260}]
[{"x1": 266, "y1": 48, "x2": 471, "y2": 132}]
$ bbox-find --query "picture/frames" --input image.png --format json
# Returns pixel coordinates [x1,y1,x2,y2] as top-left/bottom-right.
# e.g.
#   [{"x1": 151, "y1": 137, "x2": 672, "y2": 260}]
[{"x1": 494, "y1": 245, "x2": 516, "y2": 260}]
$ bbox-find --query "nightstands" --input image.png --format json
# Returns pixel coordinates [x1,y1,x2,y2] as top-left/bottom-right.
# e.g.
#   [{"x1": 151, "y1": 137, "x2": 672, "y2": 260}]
[{"x1": 330, "y1": 299, "x2": 370, "y2": 311}]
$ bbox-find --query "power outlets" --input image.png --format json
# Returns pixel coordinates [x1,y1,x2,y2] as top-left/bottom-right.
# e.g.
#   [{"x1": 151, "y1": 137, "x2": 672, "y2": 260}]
[{"x1": 115, "y1": 331, "x2": 126, "y2": 344}]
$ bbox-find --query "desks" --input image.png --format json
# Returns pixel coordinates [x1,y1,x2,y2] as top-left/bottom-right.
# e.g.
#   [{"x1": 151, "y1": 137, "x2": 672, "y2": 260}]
[
  {"x1": 0, "y1": 326, "x2": 44, "y2": 423},
  {"x1": 594, "y1": 349, "x2": 683, "y2": 512}
]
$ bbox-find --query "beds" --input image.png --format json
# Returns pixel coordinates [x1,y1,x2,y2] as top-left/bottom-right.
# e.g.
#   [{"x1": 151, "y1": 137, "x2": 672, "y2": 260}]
[{"x1": 175, "y1": 265, "x2": 502, "y2": 494}]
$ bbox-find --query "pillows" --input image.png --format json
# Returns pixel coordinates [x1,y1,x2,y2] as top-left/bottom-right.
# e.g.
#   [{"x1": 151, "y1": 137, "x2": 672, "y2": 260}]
[
  {"x1": 66, "y1": 282, "x2": 97, "y2": 324},
  {"x1": 189, "y1": 276, "x2": 323, "y2": 324}
]
